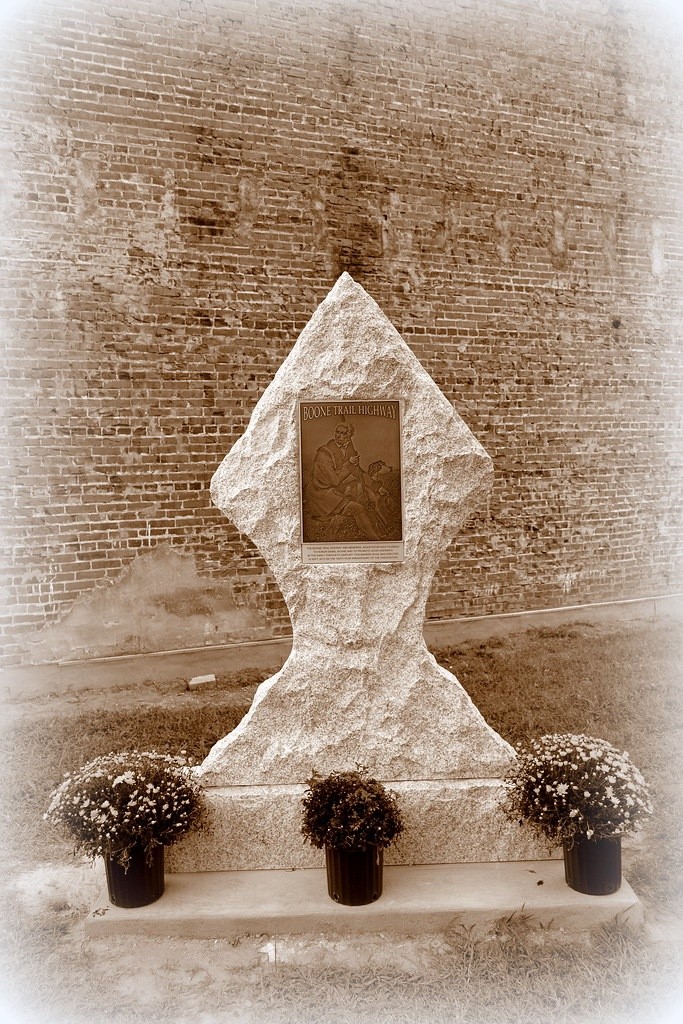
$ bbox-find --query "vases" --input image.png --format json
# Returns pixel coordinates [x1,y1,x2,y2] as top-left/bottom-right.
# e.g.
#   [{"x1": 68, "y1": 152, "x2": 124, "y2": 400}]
[
  {"x1": 102, "y1": 844, "x2": 165, "y2": 909},
  {"x1": 561, "y1": 833, "x2": 622, "y2": 895},
  {"x1": 324, "y1": 845, "x2": 383, "y2": 907}
]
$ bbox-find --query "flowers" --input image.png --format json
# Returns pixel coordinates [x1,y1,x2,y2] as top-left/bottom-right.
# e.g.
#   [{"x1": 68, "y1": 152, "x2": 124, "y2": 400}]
[
  {"x1": 493, "y1": 732, "x2": 654, "y2": 855},
  {"x1": 44, "y1": 748, "x2": 216, "y2": 875},
  {"x1": 299, "y1": 762, "x2": 407, "y2": 852}
]
